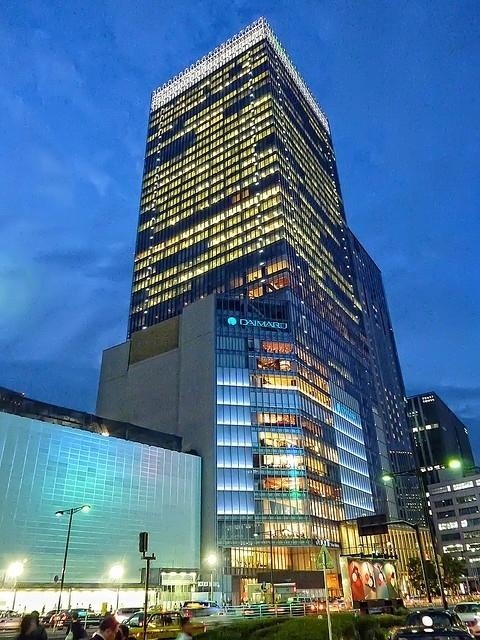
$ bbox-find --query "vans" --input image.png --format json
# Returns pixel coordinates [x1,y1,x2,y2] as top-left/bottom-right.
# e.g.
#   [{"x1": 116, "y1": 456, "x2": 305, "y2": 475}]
[{"x1": 181, "y1": 600, "x2": 224, "y2": 617}]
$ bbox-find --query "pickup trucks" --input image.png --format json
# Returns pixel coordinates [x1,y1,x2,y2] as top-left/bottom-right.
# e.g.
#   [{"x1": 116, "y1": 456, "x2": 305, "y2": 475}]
[{"x1": 121, "y1": 611, "x2": 208, "y2": 640}]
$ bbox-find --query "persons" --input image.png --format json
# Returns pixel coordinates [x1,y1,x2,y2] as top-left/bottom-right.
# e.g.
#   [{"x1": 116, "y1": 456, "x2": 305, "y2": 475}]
[
  {"x1": 175, "y1": 617, "x2": 193, "y2": 640},
  {"x1": 174, "y1": 602, "x2": 182, "y2": 611},
  {"x1": 14, "y1": 610, "x2": 137, "y2": 640}
]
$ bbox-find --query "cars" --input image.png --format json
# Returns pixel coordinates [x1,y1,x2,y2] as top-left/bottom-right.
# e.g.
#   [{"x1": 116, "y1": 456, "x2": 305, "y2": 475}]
[
  {"x1": 40, "y1": 609, "x2": 102, "y2": 627},
  {"x1": 0, "y1": 609, "x2": 24, "y2": 631},
  {"x1": 386, "y1": 600, "x2": 480, "y2": 639}
]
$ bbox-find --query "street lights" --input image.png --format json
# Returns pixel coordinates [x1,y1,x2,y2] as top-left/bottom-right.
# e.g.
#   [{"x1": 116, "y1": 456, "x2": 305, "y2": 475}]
[
  {"x1": 252, "y1": 528, "x2": 290, "y2": 607},
  {"x1": 380, "y1": 458, "x2": 462, "y2": 610},
  {"x1": 53, "y1": 504, "x2": 91, "y2": 615}
]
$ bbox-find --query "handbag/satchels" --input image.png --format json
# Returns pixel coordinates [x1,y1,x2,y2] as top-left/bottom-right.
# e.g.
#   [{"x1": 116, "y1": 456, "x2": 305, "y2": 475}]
[{"x1": 65, "y1": 624, "x2": 74, "y2": 640}]
